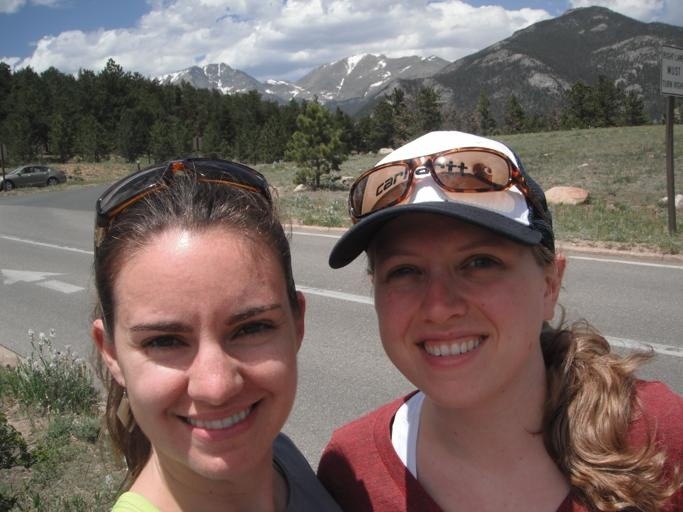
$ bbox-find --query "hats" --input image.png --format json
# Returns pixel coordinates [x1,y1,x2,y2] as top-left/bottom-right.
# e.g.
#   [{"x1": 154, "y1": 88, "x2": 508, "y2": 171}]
[{"x1": 329, "y1": 130, "x2": 554, "y2": 269}]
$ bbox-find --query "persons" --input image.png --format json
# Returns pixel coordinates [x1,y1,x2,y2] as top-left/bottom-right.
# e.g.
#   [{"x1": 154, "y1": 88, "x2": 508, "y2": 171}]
[
  {"x1": 317, "y1": 131, "x2": 683, "y2": 512},
  {"x1": 91, "y1": 158, "x2": 343, "y2": 512}
]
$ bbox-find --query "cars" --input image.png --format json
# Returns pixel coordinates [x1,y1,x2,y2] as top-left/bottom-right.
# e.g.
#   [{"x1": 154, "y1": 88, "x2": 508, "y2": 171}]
[{"x1": 0, "y1": 164, "x2": 67, "y2": 191}]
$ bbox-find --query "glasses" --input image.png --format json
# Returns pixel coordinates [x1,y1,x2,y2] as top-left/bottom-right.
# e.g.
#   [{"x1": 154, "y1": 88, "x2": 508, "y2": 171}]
[
  {"x1": 349, "y1": 147, "x2": 550, "y2": 224},
  {"x1": 94, "y1": 159, "x2": 274, "y2": 260}
]
